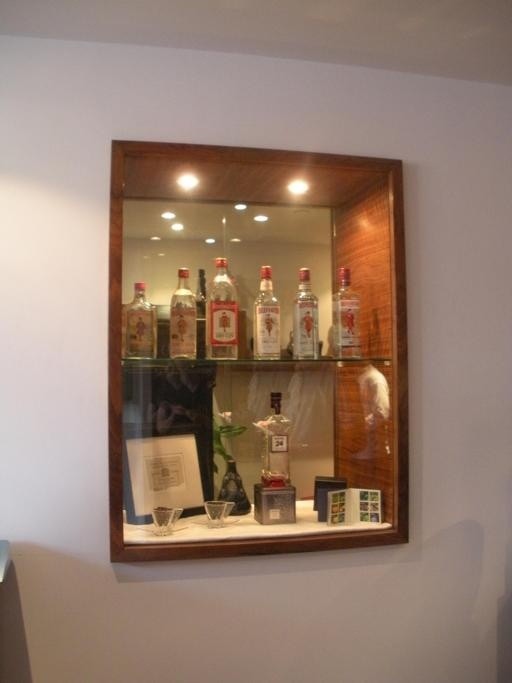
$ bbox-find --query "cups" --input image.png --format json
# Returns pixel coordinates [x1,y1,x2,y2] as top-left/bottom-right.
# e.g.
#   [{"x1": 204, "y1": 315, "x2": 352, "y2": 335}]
[
  {"x1": 149, "y1": 507, "x2": 184, "y2": 535},
  {"x1": 203, "y1": 501, "x2": 235, "y2": 528}
]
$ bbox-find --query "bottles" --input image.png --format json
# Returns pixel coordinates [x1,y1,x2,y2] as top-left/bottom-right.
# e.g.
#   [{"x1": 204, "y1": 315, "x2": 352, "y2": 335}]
[
  {"x1": 118, "y1": 256, "x2": 360, "y2": 359},
  {"x1": 262, "y1": 392, "x2": 292, "y2": 489}
]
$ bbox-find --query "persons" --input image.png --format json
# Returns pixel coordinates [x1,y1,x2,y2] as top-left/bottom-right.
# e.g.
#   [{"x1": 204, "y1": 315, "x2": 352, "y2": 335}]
[{"x1": 123, "y1": 324, "x2": 393, "y2": 504}]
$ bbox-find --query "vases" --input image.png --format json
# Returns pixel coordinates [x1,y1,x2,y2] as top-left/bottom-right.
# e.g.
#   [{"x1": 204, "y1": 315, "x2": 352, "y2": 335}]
[{"x1": 216, "y1": 462, "x2": 251, "y2": 516}]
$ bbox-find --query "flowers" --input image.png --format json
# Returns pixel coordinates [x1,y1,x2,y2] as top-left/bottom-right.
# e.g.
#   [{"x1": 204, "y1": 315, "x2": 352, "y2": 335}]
[{"x1": 212, "y1": 411, "x2": 248, "y2": 474}]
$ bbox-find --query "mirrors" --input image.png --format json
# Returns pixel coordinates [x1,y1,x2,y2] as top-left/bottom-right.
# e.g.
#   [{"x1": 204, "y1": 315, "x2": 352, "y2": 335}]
[{"x1": 111, "y1": 139, "x2": 410, "y2": 562}]
